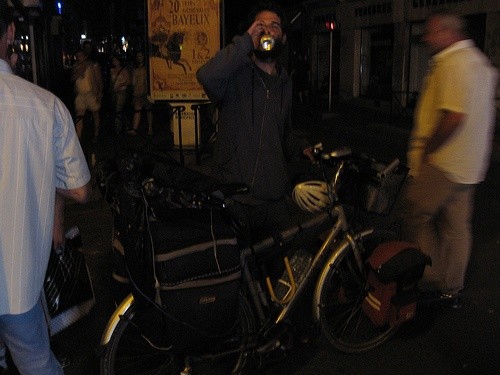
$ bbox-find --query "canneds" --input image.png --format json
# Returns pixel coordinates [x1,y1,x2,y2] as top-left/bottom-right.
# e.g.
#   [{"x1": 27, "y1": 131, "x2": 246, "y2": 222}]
[{"x1": 259, "y1": 32, "x2": 273, "y2": 52}]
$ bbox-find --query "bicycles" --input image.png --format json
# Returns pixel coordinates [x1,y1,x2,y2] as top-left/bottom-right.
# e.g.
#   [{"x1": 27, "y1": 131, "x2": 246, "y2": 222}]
[{"x1": 98, "y1": 142, "x2": 411, "y2": 375}]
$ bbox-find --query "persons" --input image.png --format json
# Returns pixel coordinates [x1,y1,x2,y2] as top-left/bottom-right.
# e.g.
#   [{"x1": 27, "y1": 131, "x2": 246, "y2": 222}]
[
  {"x1": 398, "y1": 9, "x2": 498, "y2": 306},
  {"x1": 197, "y1": 8, "x2": 319, "y2": 208},
  {"x1": 0, "y1": 1, "x2": 92, "y2": 375},
  {"x1": 53, "y1": 193, "x2": 65, "y2": 249},
  {"x1": 72, "y1": 41, "x2": 157, "y2": 144}
]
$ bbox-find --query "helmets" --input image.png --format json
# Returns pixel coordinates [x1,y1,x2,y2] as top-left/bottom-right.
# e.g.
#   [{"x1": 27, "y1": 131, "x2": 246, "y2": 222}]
[{"x1": 291, "y1": 180, "x2": 337, "y2": 215}]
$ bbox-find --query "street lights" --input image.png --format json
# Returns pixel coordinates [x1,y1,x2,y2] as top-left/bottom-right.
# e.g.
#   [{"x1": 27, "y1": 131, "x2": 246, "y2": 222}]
[{"x1": 324, "y1": 17, "x2": 338, "y2": 112}]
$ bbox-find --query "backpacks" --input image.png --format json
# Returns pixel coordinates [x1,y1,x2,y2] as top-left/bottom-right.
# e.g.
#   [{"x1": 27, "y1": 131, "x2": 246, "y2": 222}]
[{"x1": 148, "y1": 207, "x2": 242, "y2": 357}]
